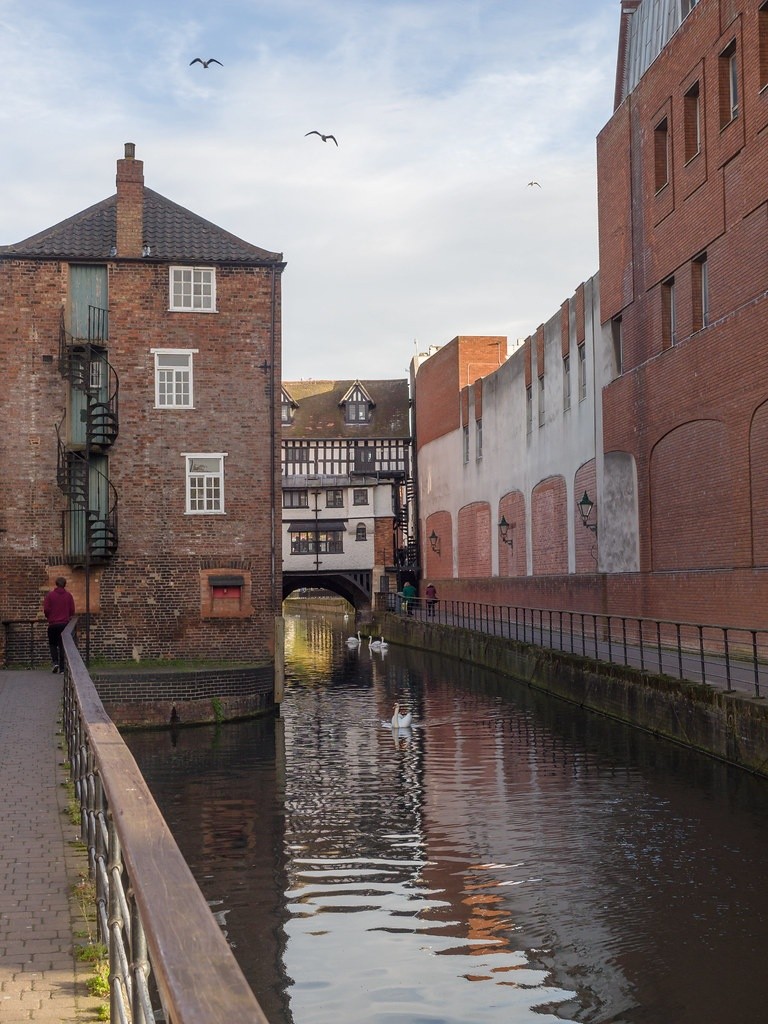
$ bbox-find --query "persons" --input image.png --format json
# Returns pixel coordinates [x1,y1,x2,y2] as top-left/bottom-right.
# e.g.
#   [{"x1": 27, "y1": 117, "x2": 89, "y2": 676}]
[
  {"x1": 44, "y1": 577, "x2": 75, "y2": 675},
  {"x1": 426, "y1": 583, "x2": 438, "y2": 615},
  {"x1": 402, "y1": 582, "x2": 417, "y2": 617}
]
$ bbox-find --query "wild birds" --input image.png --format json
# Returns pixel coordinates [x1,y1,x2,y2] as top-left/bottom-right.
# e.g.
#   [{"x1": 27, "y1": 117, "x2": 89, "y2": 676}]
[
  {"x1": 189, "y1": 57, "x2": 224, "y2": 69},
  {"x1": 526, "y1": 181, "x2": 543, "y2": 189},
  {"x1": 304, "y1": 130, "x2": 339, "y2": 147}
]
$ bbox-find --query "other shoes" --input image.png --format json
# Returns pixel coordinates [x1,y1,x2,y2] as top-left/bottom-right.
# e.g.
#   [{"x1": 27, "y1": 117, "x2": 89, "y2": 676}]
[
  {"x1": 428, "y1": 614, "x2": 435, "y2": 616},
  {"x1": 408, "y1": 613, "x2": 414, "y2": 615}
]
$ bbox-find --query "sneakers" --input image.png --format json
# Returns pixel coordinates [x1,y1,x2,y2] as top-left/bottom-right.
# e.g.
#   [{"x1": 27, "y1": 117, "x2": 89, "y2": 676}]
[
  {"x1": 60, "y1": 671, "x2": 64, "y2": 674},
  {"x1": 52, "y1": 664, "x2": 59, "y2": 673}
]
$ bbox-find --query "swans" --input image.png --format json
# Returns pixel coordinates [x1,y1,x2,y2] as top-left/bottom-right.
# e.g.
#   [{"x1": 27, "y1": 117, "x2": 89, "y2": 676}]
[
  {"x1": 343, "y1": 611, "x2": 389, "y2": 648},
  {"x1": 391, "y1": 702, "x2": 413, "y2": 728}
]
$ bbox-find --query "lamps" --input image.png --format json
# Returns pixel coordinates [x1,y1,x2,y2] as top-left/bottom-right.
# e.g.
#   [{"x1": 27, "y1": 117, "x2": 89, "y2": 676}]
[
  {"x1": 429, "y1": 529, "x2": 440, "y2": 556},
  {"x1": 499, "y1": 515, "x2": 513, "y2": 549},
  {"x1": 576, "y1": 490, "x2": 597, "y2": 536}
]
